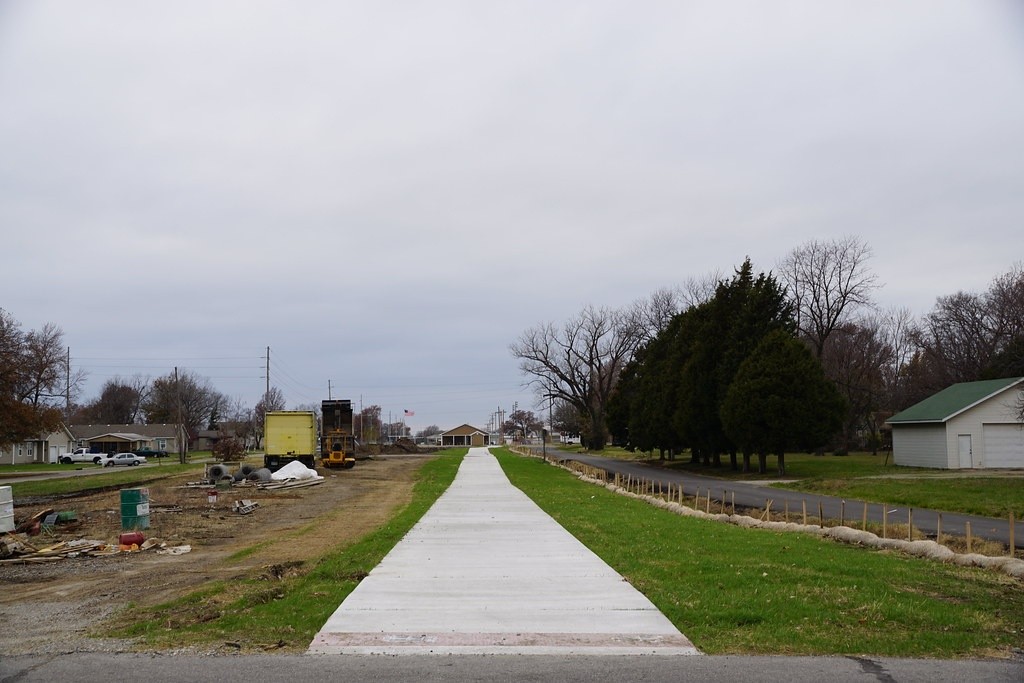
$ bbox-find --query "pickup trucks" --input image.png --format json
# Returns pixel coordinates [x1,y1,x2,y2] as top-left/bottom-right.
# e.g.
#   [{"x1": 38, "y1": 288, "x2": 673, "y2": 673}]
[
  {"x1": 132, "y1": 446, "x2": 168, "y2": 458},
  {"x1": 61, "y1": 448, "x2": 108, "y2": 464}
]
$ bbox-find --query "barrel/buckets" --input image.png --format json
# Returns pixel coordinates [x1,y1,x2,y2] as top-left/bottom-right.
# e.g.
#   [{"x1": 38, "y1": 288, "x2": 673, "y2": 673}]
[
  {"x1": 0, "y1": 486, "x2": 15, "y2": 533},
  {"x1": 118, "y1": 488, "x2": 152, "y2": 532}
]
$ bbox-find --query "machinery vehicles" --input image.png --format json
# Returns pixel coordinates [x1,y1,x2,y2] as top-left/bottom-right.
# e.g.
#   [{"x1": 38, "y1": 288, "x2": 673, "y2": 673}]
[{"x1": 320, "y1": 399, "x2": 357, "y2": 469}]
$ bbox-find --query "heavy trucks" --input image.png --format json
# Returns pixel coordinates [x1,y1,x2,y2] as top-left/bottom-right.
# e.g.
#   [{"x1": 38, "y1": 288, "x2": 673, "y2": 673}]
[{"x1": 263, "y1": 409, "x2": 318, "y2": 473}]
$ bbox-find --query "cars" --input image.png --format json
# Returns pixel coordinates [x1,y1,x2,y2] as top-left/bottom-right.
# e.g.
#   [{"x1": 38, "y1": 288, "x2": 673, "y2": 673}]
[{"x1": 101, "y1": 453, "x2": 147, "y2": 467}]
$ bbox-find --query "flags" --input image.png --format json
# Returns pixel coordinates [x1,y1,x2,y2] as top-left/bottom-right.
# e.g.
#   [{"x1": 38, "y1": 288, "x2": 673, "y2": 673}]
[{"x1": 405, "y1": 410, "x2": 414, "y2": 416}]
